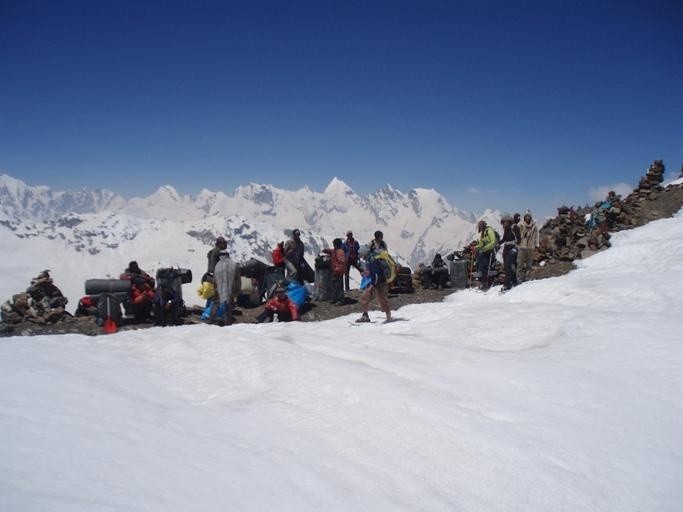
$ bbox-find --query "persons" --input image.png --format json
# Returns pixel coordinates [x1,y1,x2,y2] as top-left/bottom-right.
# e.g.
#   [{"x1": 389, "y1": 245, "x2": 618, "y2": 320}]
[
  {"x1": 131, "y1": 278, "x2": 151, "y2": 321},
  {"x1": 464, "y1": 206, "x2": 540, "y2": 295},
  {"x1": 201, "y1": 228, "x2": 395, "y2": 327},
  {"x1": 431, "y1": 253, "x2": 448, "y2": 288},
  {"x1": 603, "y1": 191, "x2": 621, "y2": 216},
  {"x1": 153, "y1": 279, "x2": 185, "y2": 326},
  {"x1": 119, "y1": 259, "x2": 155, "y2": 311},
  {"x1": 412, "y1": 263, "x2": 429, "y2": 290}
]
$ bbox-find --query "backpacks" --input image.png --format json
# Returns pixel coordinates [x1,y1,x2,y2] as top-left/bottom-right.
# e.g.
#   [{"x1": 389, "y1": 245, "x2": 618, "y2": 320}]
[{"x1": 375, "y1": 251, "x2": 399, "y2": 285}]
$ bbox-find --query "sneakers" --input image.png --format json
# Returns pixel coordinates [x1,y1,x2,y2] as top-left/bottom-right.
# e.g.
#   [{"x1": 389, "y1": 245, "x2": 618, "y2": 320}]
[
  {"x1": 354, "y1": 313, "x2": 370, "y2": 323},
  {"x1": 479, "y1": 270, "x2": 532, "y2": 292}
]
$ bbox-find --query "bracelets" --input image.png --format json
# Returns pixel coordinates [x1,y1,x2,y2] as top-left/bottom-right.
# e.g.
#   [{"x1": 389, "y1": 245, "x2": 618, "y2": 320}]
[{"x1": 167, "y1": 299, "x2": 171, "y2": 304}]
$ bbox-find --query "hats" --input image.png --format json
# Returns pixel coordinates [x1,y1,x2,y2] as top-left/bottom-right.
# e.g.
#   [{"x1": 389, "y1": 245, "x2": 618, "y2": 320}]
[
  {"x1": 217, "y1": 237, "x2": 226, "y2": 243},
  {"x1": 479, "y1": 209, "x2": 535, "y2": 226},
  {"x1": 293, "y1": 230, "x2": 300, "y2": 237},
  {"x1": 374, "y1": 230, "x2": 383, "y2": 242},
  {"x1": 347, "y1": 231, "x2": 352, "y2": 236},
  {"x1": 418, "y1": 261, "x2": 424, "y2": 267},
  {"x1": 434, "y1": 253, "x2": 442, "y2": 259},
  {"x1": 333, "y1": 238, "x2": 342, "y2": 248},
  {"x1": 357, "y1": 243, "x2": 371, "y2": 256}
]
